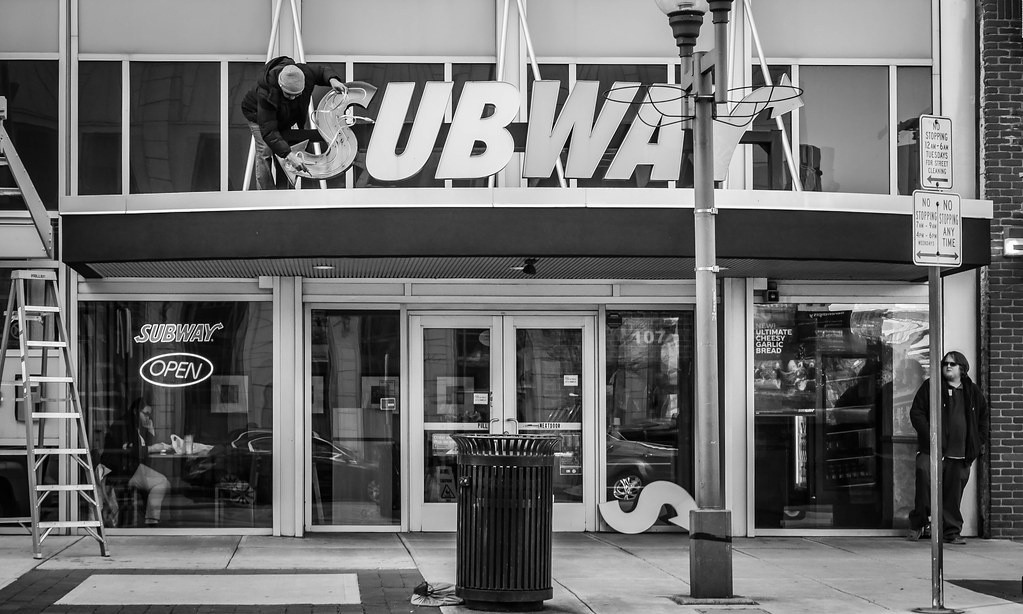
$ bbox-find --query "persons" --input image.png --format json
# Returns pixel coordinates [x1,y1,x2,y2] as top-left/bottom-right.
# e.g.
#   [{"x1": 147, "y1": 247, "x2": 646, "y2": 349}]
[
  {"x1": 906, "y1": 350, "x2": 989, "y2": 544},
  {"x1": 240, "y1": 56, "x2": 343, "y2": 190},
  {"x1": 868, "y1": 359, "x2": 928, "y2": 529},
  {"x1": 100, "y1": 397, "x2": 171, "y2": 528}
]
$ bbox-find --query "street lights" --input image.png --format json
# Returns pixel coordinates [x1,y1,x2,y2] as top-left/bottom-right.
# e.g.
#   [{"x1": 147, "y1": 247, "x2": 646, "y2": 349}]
[{"x1": 656, "y1": 0, "x2": 736, "y2": 601}]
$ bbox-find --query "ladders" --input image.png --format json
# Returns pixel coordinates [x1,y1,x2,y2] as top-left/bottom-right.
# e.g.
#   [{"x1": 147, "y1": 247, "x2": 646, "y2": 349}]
[{"x1": 0, "y1": 268, "x2": 113, "y2": 561}]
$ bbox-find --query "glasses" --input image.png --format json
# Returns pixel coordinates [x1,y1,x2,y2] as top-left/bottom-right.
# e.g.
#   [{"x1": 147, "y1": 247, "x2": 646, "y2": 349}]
[
  {"x1": 942, "y1": 362, "x2": 958, "y2": 366},
  {"x1": 284, "y1": 91, "x2": 302, "y2": 96},
  {"x1": 140, "y1": 410, "x2": 152, "y2": 417}
]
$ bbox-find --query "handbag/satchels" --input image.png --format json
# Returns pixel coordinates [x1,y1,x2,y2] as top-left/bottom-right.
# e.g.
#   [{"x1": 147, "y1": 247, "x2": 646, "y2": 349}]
[{"x1": 171, "y1": 434, "x2": 215, "y2": 455}]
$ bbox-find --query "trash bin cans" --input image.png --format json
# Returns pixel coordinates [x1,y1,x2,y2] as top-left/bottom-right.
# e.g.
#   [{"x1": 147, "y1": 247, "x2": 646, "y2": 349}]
[{"x1": 448, "y1": 432, "x2": 562, "y2": 612}]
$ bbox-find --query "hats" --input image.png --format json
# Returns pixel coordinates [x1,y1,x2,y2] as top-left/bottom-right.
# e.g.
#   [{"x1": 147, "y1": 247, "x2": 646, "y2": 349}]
[{"x1": 278, "y1": 65, "x2": 305, "y2": 94}]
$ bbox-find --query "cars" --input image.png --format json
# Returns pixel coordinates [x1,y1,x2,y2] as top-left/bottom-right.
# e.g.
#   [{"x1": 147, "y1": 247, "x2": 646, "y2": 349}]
[
  {"x1": 185, "y1": 428, "x2": 383, "y2": 508},
  {"x1": 551, "y1": 432, "x2": 678, "y2": 500}
]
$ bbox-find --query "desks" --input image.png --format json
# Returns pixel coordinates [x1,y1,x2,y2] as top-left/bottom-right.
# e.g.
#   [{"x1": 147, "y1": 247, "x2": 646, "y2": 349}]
[{"x1": 146, "y1": 448, "x2": 218, "y2": 528}]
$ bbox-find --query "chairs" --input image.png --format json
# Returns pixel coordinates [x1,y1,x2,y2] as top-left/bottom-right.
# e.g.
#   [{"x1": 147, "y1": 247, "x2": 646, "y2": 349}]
[
  {"x1": 90, "y1": 449, "x2": 140, "y2": 528},
  {"x1": 214, "y1": 451, "x2": 260, "y2": 528}
]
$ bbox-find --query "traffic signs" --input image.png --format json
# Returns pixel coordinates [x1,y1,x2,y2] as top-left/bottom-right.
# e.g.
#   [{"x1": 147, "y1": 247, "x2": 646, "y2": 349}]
[
  {"x1": 913, "y1": 191, "x2": 963, "y2": 266},
  {"x1": 919, "y1": 114, "x2": 955, "y2": 191}
]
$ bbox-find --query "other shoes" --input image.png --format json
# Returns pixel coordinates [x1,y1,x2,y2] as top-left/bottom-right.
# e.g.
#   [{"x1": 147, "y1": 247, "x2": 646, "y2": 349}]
[
  {"x1": 907, "y1": 524, "x2": 922, "y2": 541},
  {"x1": 943, "y1": 537, "x2": 967, "y2": 544}
]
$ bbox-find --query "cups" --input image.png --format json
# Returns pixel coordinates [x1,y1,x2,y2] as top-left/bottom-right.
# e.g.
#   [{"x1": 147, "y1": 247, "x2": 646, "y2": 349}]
[{"x1": 183, "y1": 435, "x2": 193, "y2": 454}]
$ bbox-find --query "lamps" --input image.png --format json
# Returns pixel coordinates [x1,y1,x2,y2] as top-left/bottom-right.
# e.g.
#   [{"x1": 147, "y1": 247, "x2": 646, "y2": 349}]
[{"x1": 523, "y1": 260, "x2": 536, "y2": 275}]
